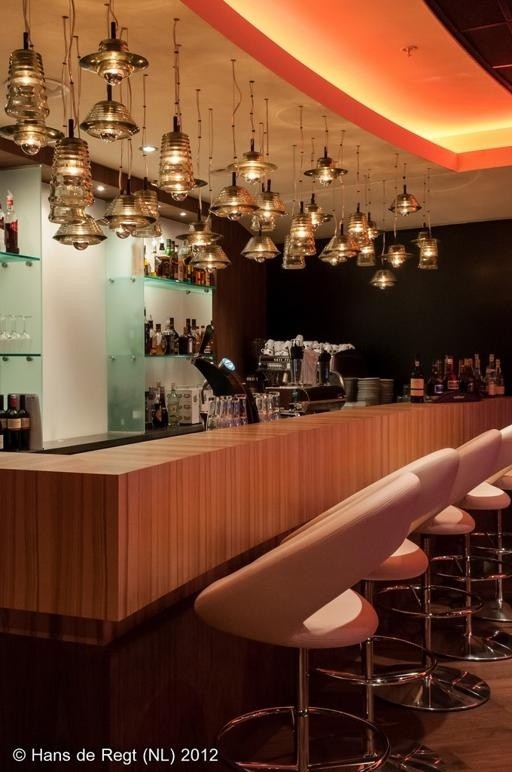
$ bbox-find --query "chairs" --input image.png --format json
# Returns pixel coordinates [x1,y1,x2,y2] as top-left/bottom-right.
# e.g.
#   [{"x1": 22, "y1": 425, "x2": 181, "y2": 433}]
[
  {"x1": 373, "y1": 428, "x2": 502, "y2": 711},
  {"x1": 194, "y1": 472, "x2": 421, "y2": 772},
  {"x1": 310, "y1": 447, "x2": 459, "y2": 772},
  {"x1": 430, "y1": 423, "x2": 512, "y2": 661},
  {"x1": 472, "y1": 463, "x2": 512, "y2": 622}
]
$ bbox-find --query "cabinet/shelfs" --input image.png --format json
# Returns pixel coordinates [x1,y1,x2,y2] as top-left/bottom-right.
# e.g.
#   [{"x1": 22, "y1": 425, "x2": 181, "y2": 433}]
[
  {"x1": 105, "y1": 227, "x2": 212, "y2": 436},
  {"x1": 0, "y1": 147, "x2": 43, "y2": 452}
]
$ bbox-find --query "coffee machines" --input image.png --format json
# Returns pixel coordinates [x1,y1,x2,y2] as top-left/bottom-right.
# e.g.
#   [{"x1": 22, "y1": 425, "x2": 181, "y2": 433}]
[{"x1": 257, "y1": 360, "x2": 346, "y2": 417}]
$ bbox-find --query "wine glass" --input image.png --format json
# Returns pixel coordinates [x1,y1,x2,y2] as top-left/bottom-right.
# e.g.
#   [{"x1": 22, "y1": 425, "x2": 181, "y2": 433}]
[{"x1": 1, "y1": 313, "x2": 33, "y2": 354}]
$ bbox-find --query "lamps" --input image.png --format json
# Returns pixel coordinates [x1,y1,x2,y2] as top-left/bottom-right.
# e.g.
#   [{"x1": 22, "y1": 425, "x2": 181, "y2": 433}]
[{"x1": 1, "y1": 0, "x2": 440, "y2": 289}]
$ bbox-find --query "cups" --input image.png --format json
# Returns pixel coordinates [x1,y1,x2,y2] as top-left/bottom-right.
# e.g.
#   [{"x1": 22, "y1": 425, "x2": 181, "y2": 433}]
[
  {"x1": 260, "y1": 334, "x2": 355, "y2": 356},
  {"x1": 289, "y1": 359, "x2": 303, "y2": 385},
  {"x1": 317, "y1": 361, "x2": 329, "y2": 385},
  {"x1": 207, "y1": 391, "x2": 281, "y2": 430}
]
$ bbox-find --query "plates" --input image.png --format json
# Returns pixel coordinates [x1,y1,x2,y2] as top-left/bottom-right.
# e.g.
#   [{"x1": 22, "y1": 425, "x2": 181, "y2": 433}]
[{"x1": 344, "y1": 377, "x2": 393, "y2": 404}]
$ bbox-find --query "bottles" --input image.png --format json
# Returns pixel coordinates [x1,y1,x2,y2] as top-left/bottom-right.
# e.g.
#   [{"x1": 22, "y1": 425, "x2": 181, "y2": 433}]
[
  {"x1": 0, "y1": 394, "x2": 32, "y2": 451},
  {"x1": 144, "y1": 237, "x2": 210, "y2": 287},
  {"x1": 146, "y1": 380, "x2": 168, "y2": 429},
  {"x1": 410, "y1": 354, "x2": 425, "y2": 402},
  {"x1": 427, "y1": 352, "x2": 504, "y2": 396},
  {"x1": 1, "y1": 203, "x2": 4, "y2": 253},
  {"x1": 168, "y1": 382, "x2": 180, "y2": 427},
  {"x1": 144, "y1": 307, "x2": 211, "y2": 355},
  {"x1": 4, "y1": 195, "x2": 19, "y2": 253}
]
